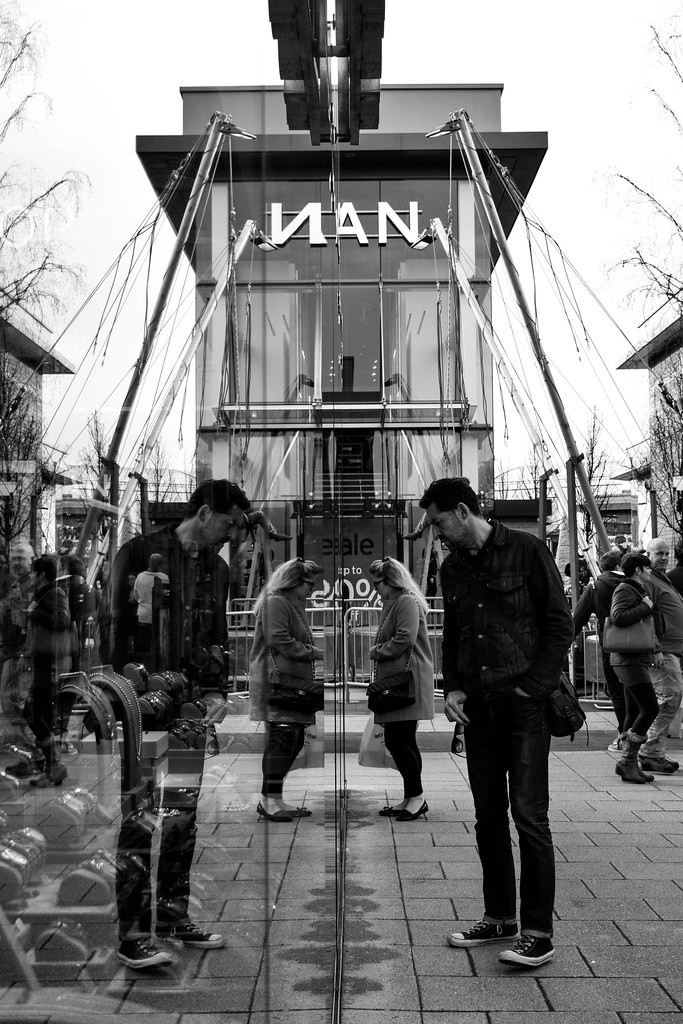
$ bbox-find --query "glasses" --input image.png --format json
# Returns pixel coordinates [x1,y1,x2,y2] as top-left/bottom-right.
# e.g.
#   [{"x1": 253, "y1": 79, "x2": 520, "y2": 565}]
[
  {"x1": 451, "y1": 720, "x2": 466, "y2": 758},
  {"x1": 204, "y1": 724, "x2": 220, "y2": 760},
  {"x1": 303, "y1": 580, "x2": 316, "y2": 590},
  {"x1": 373, "y1": 579, "x2": 386, "y2": 590}
]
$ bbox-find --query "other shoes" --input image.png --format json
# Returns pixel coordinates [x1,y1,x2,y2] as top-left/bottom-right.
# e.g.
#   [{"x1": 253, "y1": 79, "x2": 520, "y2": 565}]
[
  {"x1": 608, "y1": 743, "x2": 623, "y2": 752},
  {"x1": 636, "y1": 753, "x2": 674, "y2": 773},
  {"x1": 641, "y1": 758, "x2": 679, "y2": 771}
]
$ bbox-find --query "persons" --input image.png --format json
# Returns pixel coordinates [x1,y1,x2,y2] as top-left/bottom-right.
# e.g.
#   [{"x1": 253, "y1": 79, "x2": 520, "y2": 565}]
[
  {"x1": 251, "y1": 556, "x2": 325, "y2": 820},
  {"x1": 0, "y1": 543, "x2": 36, "y2": 778},
  {"x1": 665, "y1": 539, "x2": 683, "y2": 599},
  {"x1": 21, "y1": 556, "x2": 67, "y2": 786},
  {"x1": 113, "y1": 573, "x2": 138, "y2": 672},
  {"x1": 636, "y1": 538, "x2": 683, "y2": 773},
  {"x1": 97, "y1": 478, "x2": 252, "y2": 970},
  {"x1": 610, "y1": 552, "x2": 664, "y2": 783},
  {"x1": 368, "y1": 556, "x2": 435, "y2": 821},
  {"x1": 572, "y1": 551, "x2": 628, "y2": 751},
  {"x1": 612, "y1": 535, "x2": 631, "y2": 562},
  {"x1": 563, "y1": 559, "x2": 586, "y2": 609},
  {"x1": 60, "y1": 555, "x2": 104, "y2": 758},
  {"x1": 418, "y1": 476, "x2": 575, "y2": 967},
  {"x1": 134, "y1": 554, "x2": 170, "y2": 674},
  {"x1": 50, "y1": 540, "x2": 75, "y2": 567}
]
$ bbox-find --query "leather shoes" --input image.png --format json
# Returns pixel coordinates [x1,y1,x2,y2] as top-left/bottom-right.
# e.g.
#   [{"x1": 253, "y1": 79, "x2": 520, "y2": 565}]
[
  {"x1": 396, "y1": 800, "x2": 428, "y2": 820},
  {"x1": 379, "y1": 807, "x2": 402, "y2": 816},
  {"x1": 256, "y1": 803, "x2": 292, "y2": 821},
  {"x1": 284, "y1": 807, "x2": 312, "y2": 817}
]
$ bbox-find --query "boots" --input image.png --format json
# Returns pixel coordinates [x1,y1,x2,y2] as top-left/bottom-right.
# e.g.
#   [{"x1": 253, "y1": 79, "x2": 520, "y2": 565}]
[
  {"x1": 30, "y1": 732, "x2": 63, "y2": 787},
  {"x1": 623, "y1": 763, "x2": 654, "y2": 782},
  {"x1": 616, "y1": 728, "x2": 645, "y2": 783}
]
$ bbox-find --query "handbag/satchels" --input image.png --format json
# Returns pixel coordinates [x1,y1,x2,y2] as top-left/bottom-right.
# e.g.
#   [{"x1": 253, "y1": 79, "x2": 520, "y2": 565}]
[
  {"x1": 358, "y1": 710, "x2": 399, "y2": 771},
  {"x1": 268, "y1": 673, "x2": 324, "y2": 714},
  {"x1": 25, "y1": 588, "x2": 70, "y2": 656},
  {"x1": 289, "y1": 712, "x2": 324, "y2": 772},
  {"x1": 602, "y1": 583, "x2": 655, "y2": 653},
  {"x1": 366, "y1": 671, "x2": 414, "y2": 713},
  {"x1": 547, "y1": 671, "x2": 589, "y2": 746}
]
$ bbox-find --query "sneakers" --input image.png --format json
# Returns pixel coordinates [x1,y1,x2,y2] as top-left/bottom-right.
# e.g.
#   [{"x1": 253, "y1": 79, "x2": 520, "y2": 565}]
[
  {"x1": 499, "y1": 936, "x2": 555, "y2": 967},
  {"x1": 153, "y1": 920, "x2": 225, "y2": 950},
  {"x1": 118, "y1": 937, "x2": 171, "y2": 970},
  {"x1": 447, "y1": 920, "x2": 520, "y2": 947}
]
